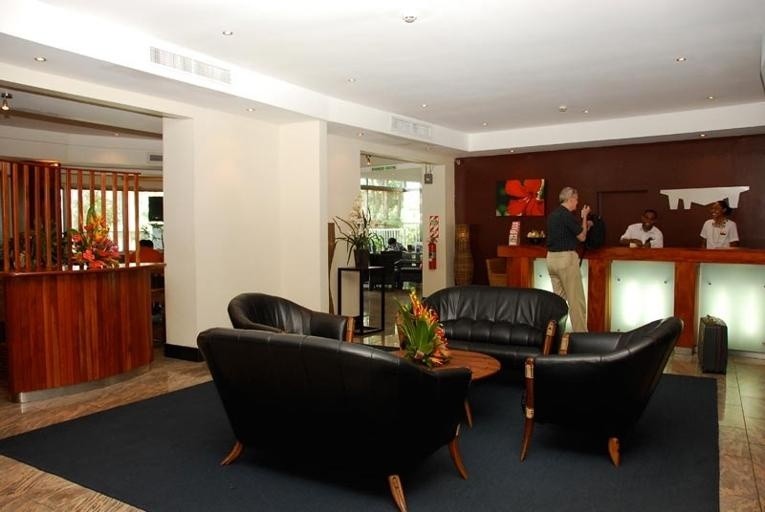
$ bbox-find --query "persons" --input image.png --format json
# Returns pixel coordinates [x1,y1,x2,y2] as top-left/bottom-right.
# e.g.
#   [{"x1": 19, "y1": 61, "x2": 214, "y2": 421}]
[
  {"x1": 545, "y1": 187, "x2": 591, "y2": 332},
  {"x1": 384, "y1": 237, "x2": 407, "y2": 251},
  {"x1": 700, "y1": 201, "x2": 739, "y2": 250},
  {"x1": 620, "y1": 209, "x2": 663, "y2": 249}
]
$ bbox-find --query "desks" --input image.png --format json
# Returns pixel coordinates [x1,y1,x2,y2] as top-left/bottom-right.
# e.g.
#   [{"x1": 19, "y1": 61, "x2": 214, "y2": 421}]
[
  {"x1": 386, "y1": 349, "x2": 501, "y2": 428},
  {"x1": 337, "y1": 266, "x2": 386, "y2": 336}
]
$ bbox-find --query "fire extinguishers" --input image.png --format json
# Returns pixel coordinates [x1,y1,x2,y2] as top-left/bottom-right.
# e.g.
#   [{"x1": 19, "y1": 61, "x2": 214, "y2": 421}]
[{"x1": 428, "y1": 237, "x2": 436, "y2": 270}]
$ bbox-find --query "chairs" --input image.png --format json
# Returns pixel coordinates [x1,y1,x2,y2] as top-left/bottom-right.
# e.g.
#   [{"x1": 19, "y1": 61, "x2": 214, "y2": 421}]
[
  {"x1": 197, "y1": 327, "x2": 474, "y2": 512},
  {"x1": 520, "y1": 316, "x2": 684, "y2": 468},
  {"x1": 369, "y1": 251, "x2": 423, "y2": 292},
  {"x1": 486, "y1": 259, "x2": 508, "y2": 288},
  {"x1": 227, "y1": 292, "x2": 354, "y2": 344},
  {"x1": 422, "y1": 286, "x2": 569, "y2": 371}
]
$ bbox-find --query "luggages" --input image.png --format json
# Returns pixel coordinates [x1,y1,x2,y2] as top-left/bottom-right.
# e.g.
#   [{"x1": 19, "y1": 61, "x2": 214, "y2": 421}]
[{"x1": 699, "y1": 315, "x2": 727, "y2": 375}]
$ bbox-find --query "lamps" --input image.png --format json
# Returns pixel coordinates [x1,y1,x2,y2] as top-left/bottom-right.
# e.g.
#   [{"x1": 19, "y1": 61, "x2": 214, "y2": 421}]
[{"x1": 1, "y1": 93, "x2": 11, "y2": 111}]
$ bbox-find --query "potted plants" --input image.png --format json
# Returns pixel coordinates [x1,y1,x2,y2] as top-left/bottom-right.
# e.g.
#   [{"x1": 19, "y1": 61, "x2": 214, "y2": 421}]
[
  {"x1": 332, "y1": 205, "x2": 384, "y2": 268},
  {"x1": 0, "y1": 231, "x2": 36, "y2": 269}
]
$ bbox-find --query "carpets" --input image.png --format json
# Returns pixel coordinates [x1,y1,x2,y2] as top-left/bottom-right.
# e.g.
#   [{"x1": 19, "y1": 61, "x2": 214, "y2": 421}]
[{"x1": 0, "y1": 345, "x2": 720, "y2": 512}]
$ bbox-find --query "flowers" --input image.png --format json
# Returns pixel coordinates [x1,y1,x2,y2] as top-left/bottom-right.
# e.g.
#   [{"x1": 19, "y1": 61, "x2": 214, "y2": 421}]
[{"x1": 63, "y1": 204, "x2": 124, "y2": 272}]
